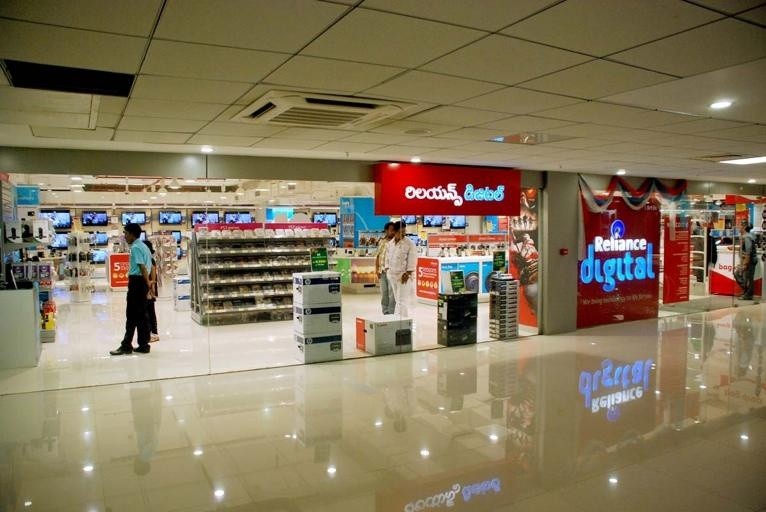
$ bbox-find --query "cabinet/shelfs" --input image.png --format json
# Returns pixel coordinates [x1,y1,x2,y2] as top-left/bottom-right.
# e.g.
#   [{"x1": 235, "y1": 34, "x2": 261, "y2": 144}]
[
  {"x1": 690, "y1": 227, "x2": 707, "y2": 285},
  {"x1": 191, "y1": 232, "x2": 337, "y2": 326}
]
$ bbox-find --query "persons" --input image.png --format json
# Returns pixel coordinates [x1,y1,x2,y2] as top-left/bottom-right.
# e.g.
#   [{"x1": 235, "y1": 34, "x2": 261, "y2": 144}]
[
  {"x1": 732, "y1": 220, "x2": 757, "y2": 301},
  {"x1": 140, "y1": 239, "x2": 160, "y2": 344},
  {"x1": 21, "y1": 224, "x2": 33, "y2": 241},
  {"x1": 375, "y1": 223, "x2": 396, "y2": 316},
  {"x1": 109, "y1": 222, "x2": 157, "y2": 355},
  {"x1": 694, "y1": 229, "x2": 717, "y2": 281},
  {"x1": 381, "y1": 222, "x2": 419, "y2": 326},
  {"x1": 377, "y1": 360, "x2": 411, "y2": 436},
  {"x1": 732, "y1": 309, "x2": 756, "y2": 379},
  {"x1": 692, "y1": 319, "x2": 716, "y2": 363},
  {"x1": 125, "y1": 364, "x2": 165, "y2": 477}
]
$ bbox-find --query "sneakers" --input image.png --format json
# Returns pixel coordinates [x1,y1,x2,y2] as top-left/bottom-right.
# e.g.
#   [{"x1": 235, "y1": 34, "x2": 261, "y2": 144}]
[{"x1": 110, "y1": 333, "x2": 158, "y2": 354}]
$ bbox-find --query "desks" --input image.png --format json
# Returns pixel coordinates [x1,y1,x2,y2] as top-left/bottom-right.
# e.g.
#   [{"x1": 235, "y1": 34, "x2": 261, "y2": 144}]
[
  {"x1": 335, "y1": 257, "x2": 381, "y2": 294},
  {"x1": 416, "y1": 256, "x2": 494, "y2": 306}
]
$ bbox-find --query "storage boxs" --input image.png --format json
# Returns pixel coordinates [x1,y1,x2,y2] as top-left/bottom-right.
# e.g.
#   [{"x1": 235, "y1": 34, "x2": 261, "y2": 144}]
[{"x1": 292, "y1": 271, "x2": 478, "y2": 364}]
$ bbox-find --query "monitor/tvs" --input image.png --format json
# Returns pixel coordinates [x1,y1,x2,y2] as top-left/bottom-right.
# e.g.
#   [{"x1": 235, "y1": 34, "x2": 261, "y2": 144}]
[
  {"x1": 40, "y1": 210, "x2": 71, "y2": 229},
  {"x1": 423, "y1": 216, "x2": 443, "y2": 227},
  {"x1": 159, "y1": 211, "x2": 182, "y2": 224},
  {"x1": 312, "y1": 212, "x2": 337, "y2": 227},
  {"x1": 121, "y1": 212, "x2": 146, "y2": 225},
  {"x1": 224, "y1": 211, "x2": 251, "y2": 224},
  {"x1": 191, "y1": 211, "x2": 219, "y2": 228},
  {"x1": 138, "y1": 231, "x2": 146, "y2": 241},
  {"x1": 81, "y1": 211, "x2": 108, "y2": 226},
  {"x1": 89, "y1": 249, "x2": 105, "y2": 264},
  {"x1": 48, "y1": 232, "x2": 71, "y2": 250},
  {"x1": 14, "y1": 249, "x2": 23, "y2": 262},
  {"x1": 447, "y1": 216, "x2": 465, "y2": 229},
  {"x1": 400, "y1": 215, "x2": 417, "y2": 224},
  {"x1": 172, "y1": 230, "x2": 181, "y2": 244},
  {"x1": 177, "y1": 246, "x2": 181, "y2": 259},
  {"x1": 89, "y1": 232, "x2": 108, "y2": 246},
  {"x1": 328, "y1": 238, "x2": 335, "y2": 247}
]
